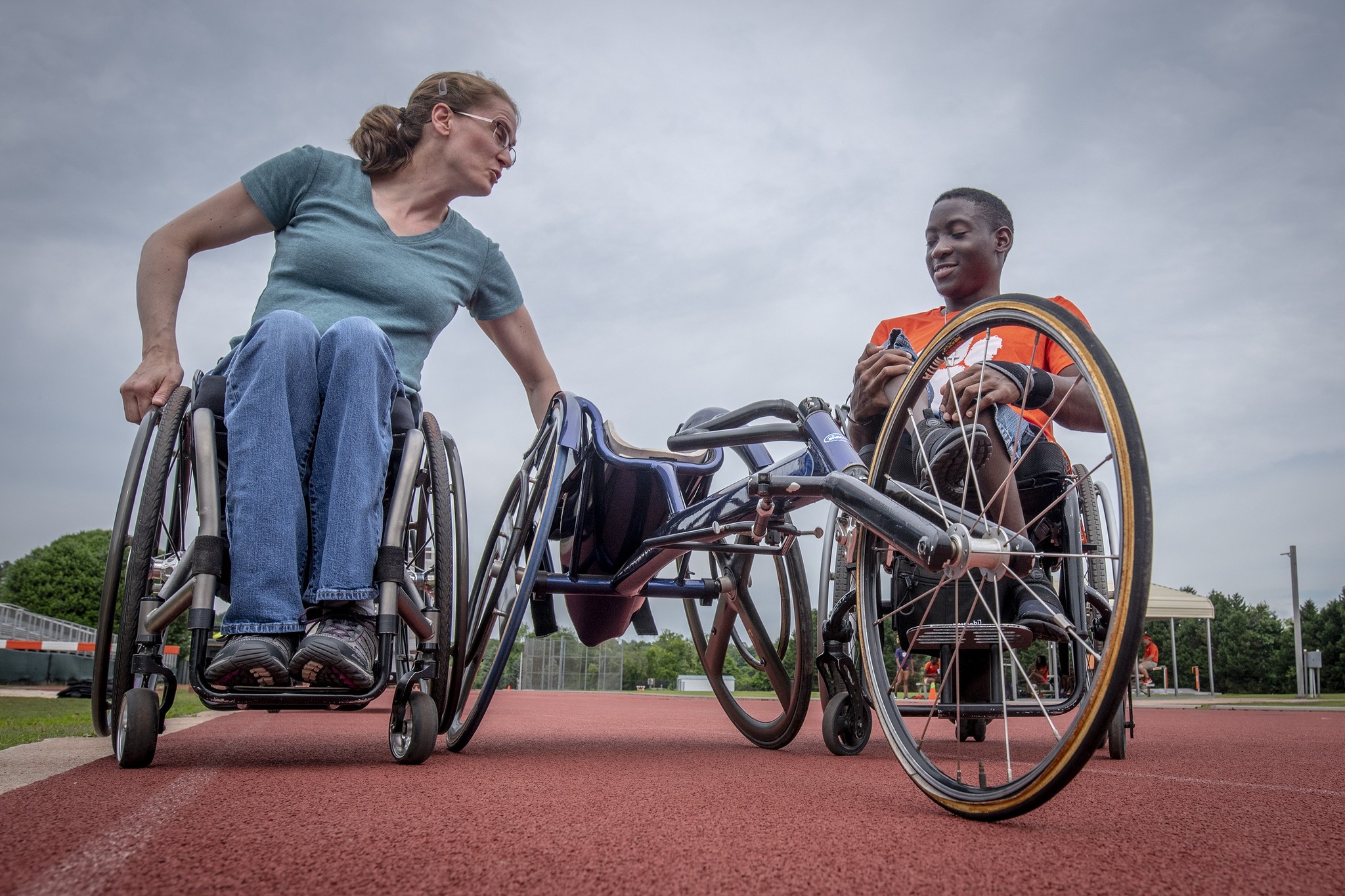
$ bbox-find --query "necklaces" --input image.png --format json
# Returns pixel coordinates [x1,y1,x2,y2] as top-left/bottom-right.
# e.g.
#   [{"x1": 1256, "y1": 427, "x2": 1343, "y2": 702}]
[{"x1": 945, "y1": 306, "x2": 960, "y2": 366}]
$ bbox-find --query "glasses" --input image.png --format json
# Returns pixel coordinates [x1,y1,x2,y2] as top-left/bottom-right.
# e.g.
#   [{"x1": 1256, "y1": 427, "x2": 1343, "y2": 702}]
[{"x1": 425, "y1": 108, "x2": 517, "y2": 170}]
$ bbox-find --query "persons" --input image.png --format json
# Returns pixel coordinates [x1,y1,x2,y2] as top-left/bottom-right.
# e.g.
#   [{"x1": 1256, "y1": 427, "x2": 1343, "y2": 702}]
[
  {"x1": 118, "y1": 72, "x2": 564, "y2": 691},
  {"x1": 845, "y1": 186, "x2": 1106, "y2": 642},
  {"x1": 1021, "y1": 655, "x2": 1049, "y2": 698},
  {"x1": 923, "y1": 656, "x2": 940, "y2": 684},
  {"x1": 894, "y1": 647, "x2": 914, "y2": 699},
  {"x1": 1132, "y1": 634, "x2": 1158, "y2": 685}
]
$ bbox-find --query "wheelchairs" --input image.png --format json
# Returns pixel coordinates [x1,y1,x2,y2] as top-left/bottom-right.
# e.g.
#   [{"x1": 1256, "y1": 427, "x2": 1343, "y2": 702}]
[
  {"x1": 814, "y1": 462, "x2": 1138, "y2": 761},
  {"x1": 91, "y1": 366, "x2": 470, "y2": 770},
  {"x1": 445, "y1": 291, "x2": 1155, "y2": 825}
]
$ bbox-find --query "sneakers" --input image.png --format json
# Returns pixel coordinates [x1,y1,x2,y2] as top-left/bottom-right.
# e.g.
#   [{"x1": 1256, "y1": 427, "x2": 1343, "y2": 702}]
[
  {"x1": 912, "y1": 419, "x2": 993, "y2": 500},
  {"x1": 205, "y1": 635, "x2": 293, "y2": 689},
  {"x1": 1142, "y1": 678, "x2": 1153, "y2": 685},
  {"x1": 290, "y1": 600, "x2": 377, "y2": 690},
  {"x1": 1132, "y1": 679, "x2": 1143, "y2": 684},
  {"x1": 1010, "y1": 568, "x2": 1071, "y2": 643}
]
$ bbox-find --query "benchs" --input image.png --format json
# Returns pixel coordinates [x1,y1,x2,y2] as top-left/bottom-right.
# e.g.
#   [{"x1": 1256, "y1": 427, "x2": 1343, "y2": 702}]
[{"x1": 916, "y1": 683, "x2": 940, "y2": 699}]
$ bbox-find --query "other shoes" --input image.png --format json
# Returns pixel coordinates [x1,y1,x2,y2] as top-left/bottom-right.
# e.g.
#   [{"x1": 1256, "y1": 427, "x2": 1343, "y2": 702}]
[{"x1": 893, "y1": 692, "x2": 897, "y2": 699}]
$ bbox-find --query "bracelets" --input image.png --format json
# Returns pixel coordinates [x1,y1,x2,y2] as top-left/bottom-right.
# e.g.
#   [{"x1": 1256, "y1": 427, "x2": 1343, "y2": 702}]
[{"x1": 845, "y1": 393, "x2": 877, "y2": 426}]
